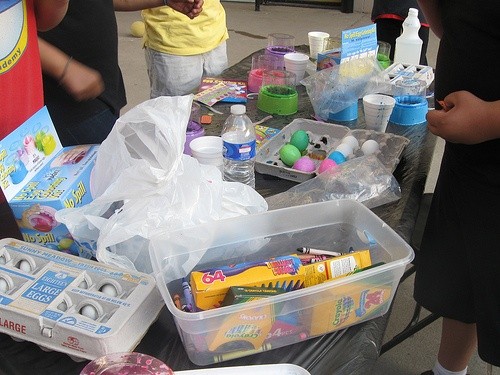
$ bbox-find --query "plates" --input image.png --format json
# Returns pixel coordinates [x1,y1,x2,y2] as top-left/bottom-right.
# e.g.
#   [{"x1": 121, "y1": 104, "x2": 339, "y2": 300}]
[{"x1": 80, "y1": 352, "x2": 174, "y2": 375}]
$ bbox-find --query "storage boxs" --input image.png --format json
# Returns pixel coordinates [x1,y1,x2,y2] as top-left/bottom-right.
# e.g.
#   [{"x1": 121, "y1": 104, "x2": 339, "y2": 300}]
[
  {"x1": 148, "y1": 198, "x2": 415, "y2": 366},
  {"x1": 0, "y1": 105, "x2": 97, "y2": 261},
  {"x1": 316, "y1": 23, "x2": 380, "y2": 80}
]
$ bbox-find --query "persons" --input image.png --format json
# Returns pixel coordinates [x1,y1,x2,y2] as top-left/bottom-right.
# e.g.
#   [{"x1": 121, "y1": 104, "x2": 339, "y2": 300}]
[
  {"x1": 412, "y1": 0, "x2": 500, "y2": 375},
  {"x1": 0, "y1": 0, "x2": 69, "y2": 243},
  {"x1": 141, "y1": 0, "x2": 229, "y2": 99},
  {"x1": 371, "y1": 0, "x2": 430, "y2": 71},
  {"x1": 38, "y1": 0, "x2": 204, "y2": 147}
]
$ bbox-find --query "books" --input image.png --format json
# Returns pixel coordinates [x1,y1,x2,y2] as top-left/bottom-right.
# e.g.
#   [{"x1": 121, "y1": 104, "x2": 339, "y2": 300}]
[{"x1": 199, "y1": 77, "x2": 248, "y2": 103}]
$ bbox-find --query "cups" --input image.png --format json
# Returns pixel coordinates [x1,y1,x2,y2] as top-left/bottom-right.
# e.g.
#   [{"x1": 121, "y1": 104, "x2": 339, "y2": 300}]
[
  {"x1": 284, "y1": 53, "x2": 309, "y2": 85},
  {"x1": 190, "y1": 136, "x2": 224, "y2": 178},
  {"x1": 362, "y1": 94, "x2": 396, "y2": 132},
  {"x1": 308, "y1": 31, "x2": 329, "y2": 59}
]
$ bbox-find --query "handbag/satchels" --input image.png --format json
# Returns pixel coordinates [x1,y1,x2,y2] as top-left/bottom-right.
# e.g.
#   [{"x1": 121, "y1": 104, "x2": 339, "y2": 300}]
[{"x1": 54, "y1": 93, "x2": 271, "y2": 283}]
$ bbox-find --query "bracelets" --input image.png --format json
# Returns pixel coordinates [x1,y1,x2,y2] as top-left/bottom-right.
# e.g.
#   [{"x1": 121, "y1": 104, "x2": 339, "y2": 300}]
[
  {"x1": 56, "y1": 56, "x2": 72, "y2": 83},
  {"x1": 164, "y1": 0, "x2": 166, "y2": 5}
]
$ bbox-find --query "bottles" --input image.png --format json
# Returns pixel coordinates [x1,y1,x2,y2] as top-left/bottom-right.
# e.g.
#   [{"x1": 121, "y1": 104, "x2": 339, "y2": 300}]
[
  {"x1": 394, "y1": 8, "x2": 423, "y2": 70},
  {"x1": 221, "y1": 104, "x2": 256, "y2": 190}
]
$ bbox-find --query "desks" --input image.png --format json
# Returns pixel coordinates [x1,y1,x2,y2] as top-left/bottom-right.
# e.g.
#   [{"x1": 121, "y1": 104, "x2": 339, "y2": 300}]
[{"x1": 130, "y1": 44, "x2": 445, "y2": 375}]
